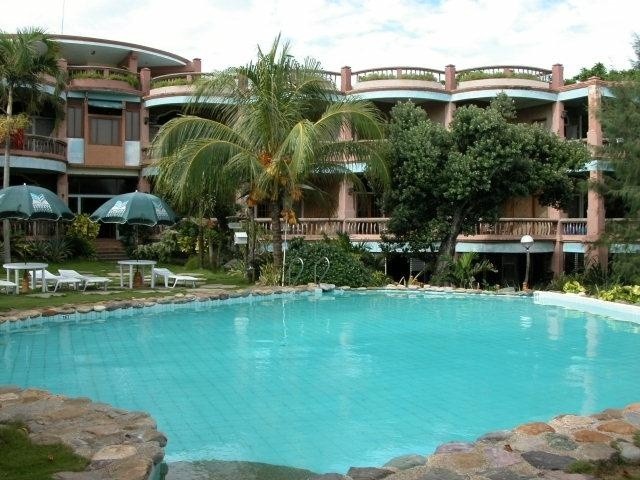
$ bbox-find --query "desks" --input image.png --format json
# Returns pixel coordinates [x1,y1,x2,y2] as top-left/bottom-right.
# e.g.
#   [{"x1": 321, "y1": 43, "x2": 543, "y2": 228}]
[
  {"x1": 2, "y1": 262, "x2": 49, "y2": 295},
  {"x1": 117, "y1": 259, "x2": 158, "y2": 290}
]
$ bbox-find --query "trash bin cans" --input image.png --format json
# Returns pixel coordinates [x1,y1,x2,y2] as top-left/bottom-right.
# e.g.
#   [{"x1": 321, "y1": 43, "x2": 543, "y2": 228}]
[{"x1": 246, "y1": 268, "x2": 255, "y2": 284}]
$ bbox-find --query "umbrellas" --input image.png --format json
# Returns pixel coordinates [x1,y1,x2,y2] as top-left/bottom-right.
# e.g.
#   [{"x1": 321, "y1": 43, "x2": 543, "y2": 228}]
[
  {"x1": 0, "y1": 183, "x2": 76, "y2": 273},
  {"x1": 88, "y1": 189, "x2": 179, "y2": 272}
]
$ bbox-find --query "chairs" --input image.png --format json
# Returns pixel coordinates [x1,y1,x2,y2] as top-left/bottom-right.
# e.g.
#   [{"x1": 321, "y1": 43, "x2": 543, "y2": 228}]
[
  {"x1": 57, "y1": 269, "x2": 113, "y2": 294},
  {"x1": 152, "y1": 267, "x2": 206, "y2": 290},
  {"x1": 28, "y1": 269, "x2": 82, "y2": 294},
  {"x1": 0, "y1": 279, "x2": 18, "y2": 296}
]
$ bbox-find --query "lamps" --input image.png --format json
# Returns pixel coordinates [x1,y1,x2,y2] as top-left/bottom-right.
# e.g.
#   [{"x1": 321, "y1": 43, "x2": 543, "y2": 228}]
[
  {"x1": 561, "y1": 110, "x2": 568, "y2": 118},
  {"x1": 144, "y1": 116, "x2": 149, "y2": 125}
]
